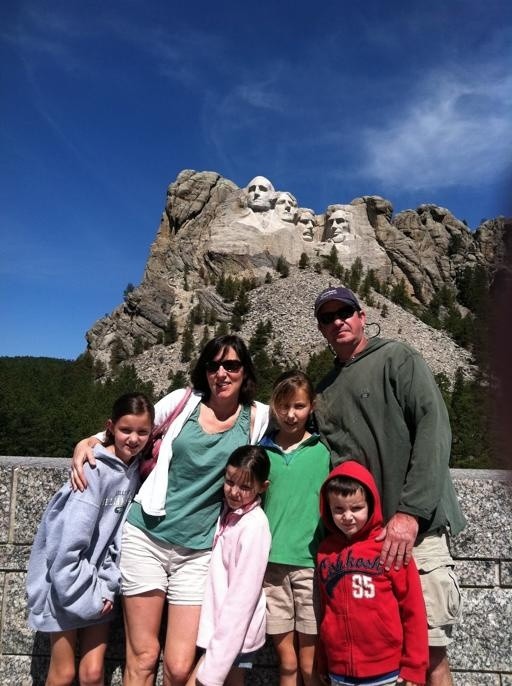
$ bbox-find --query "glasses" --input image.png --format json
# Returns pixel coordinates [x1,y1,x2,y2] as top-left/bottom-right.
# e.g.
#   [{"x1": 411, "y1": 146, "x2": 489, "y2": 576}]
[
  {"x1": 320, "y1": 307, "x2": 354, "y2": 323},
  {"x1": 206, "y1": 360, "x2": 243, "y2": 372}
]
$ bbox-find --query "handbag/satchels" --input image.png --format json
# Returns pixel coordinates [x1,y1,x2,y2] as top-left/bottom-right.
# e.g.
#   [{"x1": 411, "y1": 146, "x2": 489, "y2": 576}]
[{"x1": 140, "y1": 425, "x2": 163, "y2": 480}]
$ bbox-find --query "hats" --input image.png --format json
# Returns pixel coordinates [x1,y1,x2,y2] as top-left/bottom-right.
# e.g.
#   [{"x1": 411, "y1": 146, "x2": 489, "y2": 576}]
[{"x1": 315, "y1": 287, "x2": 361, "y2": 315}]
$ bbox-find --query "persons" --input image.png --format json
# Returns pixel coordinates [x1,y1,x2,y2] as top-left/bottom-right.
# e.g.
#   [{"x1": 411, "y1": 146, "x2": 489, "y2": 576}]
[
  {"x1": 276, "y1": 191, "x2": 299, "y2": 222},
  {"x1": 24, "y1": 391, "x2": 155, "y2": 686},
  {"x1": 311, "y1": 288, "x2": 466, "y2": 686},
  {"x1": 246, "y1": 176, "x2": 277, "y2": 211},
  {"x1": 315, "y1": 460, "x2": 431, "y2": 686},
  {"x1": 297, "y1": 207, "x2": 316, "y2": 241},
  {"x1": 257, "y1": 370, "x2": 332, "y2": 686},
  {"x1": 71, "y1": 336, "x2": 271, "y2": 686},
  {"x1": 184, "y1": 445, "x2": 272, "y2": 686},
  {"x1": 326, "y1": 204, "x2": 355, "y2": 242}
]
